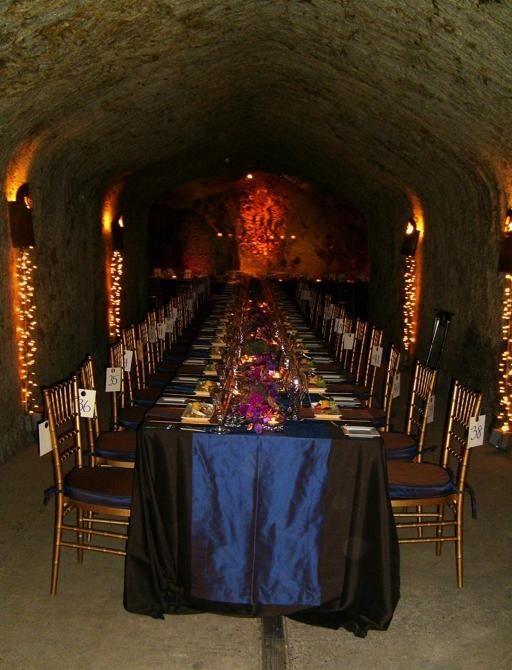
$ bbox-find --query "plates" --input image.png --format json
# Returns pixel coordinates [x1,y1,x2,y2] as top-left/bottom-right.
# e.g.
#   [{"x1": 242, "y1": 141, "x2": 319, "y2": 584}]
[
  {"x1": 341, "y1": 424, "x2": 381, "y2": 439},
  {"x1": 180, "y1": 402, "x2": 214, "y2": 421},
  {"x1": 287, "y1": 313, "x2": 330, "y2": 356},
  {"x1": 307, "y1": 387, "x2": 326, "y2": 396},
  {"x1": 320, "y1": 374, "x2": 347, "y2": 383},
  {"x1": 312, "y1": 357, "x2": 333, "y2": 365},
  {"x1": 197, "y1": 294, "x2": 230, "y2": 341},
  {"x1": 330, "y1": 395, "x2": 362, "y2": 406},
  {"x1": 193, "y1": 344, "x2": 212, "y2": 351},
  {"x1": 172, "y1": 375, "x2": 200, "y2": 385},
  {"x1": 183, "y1": 358, "x2": 207, "y2": 367},
  {"x1": 310, "y1": 402, "x2": 345, "y2": 419},
  {"x1": 193, "y1": 390, "x2": 211, "y2": 396},
  {"x1": 155, "y1": 396, "x2": 189, "y2": 406}
]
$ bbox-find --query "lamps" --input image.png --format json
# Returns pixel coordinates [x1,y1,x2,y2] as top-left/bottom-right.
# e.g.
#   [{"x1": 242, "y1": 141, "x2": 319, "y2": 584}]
[
  {"x1": 8, "y1": 182, "x2": 36, "y2": 248},
  {"x1": 111, "y1": 216, "x2": 125, "y2": 248},
  {"x1": 498, "y1": 221, "x2": 512, "y2": 274},
  {"x1": 401, "y1": 223, "x2": 418, "y2": 255}
]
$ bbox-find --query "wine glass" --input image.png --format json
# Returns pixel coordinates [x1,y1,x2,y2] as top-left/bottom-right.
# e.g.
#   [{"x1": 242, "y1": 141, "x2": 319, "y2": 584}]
[
  {"x1": 209, "y1": 328, "x2": 243, "y2": 435},
  {"x1": 270, "y1": 318, "x2": 308, "y2": 423}
]
